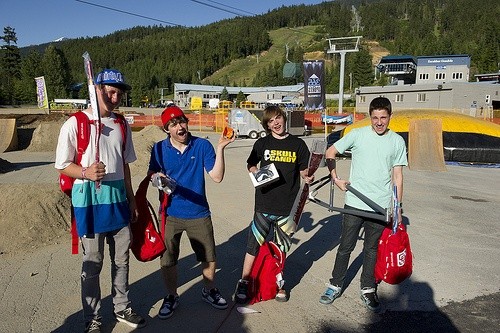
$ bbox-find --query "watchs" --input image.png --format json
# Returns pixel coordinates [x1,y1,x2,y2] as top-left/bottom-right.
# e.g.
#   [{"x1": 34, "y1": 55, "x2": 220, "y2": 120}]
[{"x1": 391, "y1": 202, "x2": 402, "y2": 208}]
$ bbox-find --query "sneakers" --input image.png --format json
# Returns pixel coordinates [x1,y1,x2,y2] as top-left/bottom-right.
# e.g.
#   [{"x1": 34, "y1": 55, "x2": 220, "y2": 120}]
[
  {"x1": 84, "y1": 318, "x2": 103, "y2": 333},
  {"x1": 234, "y1": 279, "x2": 250, "y2": 306},
  {"x1": 201, "y1": 287, "x2": 228, "y2": 309},
  {"x1": 274, "y1": 287, "x2": 287, "y2": 302},
  {"x1": 113, "y1": 308, "x2": 146, "y2": 328},
  {"x1": 158, "y1": 294, "x2": 180, "y2": 318},
  {"x1": 360, "y1": 286, "x2": 380, "y2": 313},
  {"x1": 319, "y1": 284, "x2": 344, "y2": 304}
]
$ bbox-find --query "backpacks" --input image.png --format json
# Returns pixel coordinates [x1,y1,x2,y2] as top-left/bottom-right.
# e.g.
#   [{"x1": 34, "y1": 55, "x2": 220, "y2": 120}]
[
  {"x1": 131, "y1": 172, "x2": 168, "y2": 262},
  {"x1": 250, "y1": 241, "x2": 286, "y2": 304},
  {"x1": 373, "y1": 223, "x2": 413, "y2": 285},
  {"x1": 59, "y1": 110, "x2": 126, "y2": 195}
]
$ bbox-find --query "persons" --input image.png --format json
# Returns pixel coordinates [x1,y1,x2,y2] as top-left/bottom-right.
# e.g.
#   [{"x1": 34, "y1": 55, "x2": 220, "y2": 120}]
[
  {"x1": 147, "y1": 106, "x2": 236, "y2": 320},
  {"x1": 234, "y1": 106, "x2": 314, "y2": 305},
  {"x1": 55, "y1": 70, "x2": 147, "y2": 333},
  {"x1": 320, "y1": 97, "x2": 408, "y2": 312}
]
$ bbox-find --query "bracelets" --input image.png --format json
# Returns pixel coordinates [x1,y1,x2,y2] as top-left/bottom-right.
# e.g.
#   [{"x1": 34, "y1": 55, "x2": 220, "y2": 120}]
[{"x1": 82, "y1": 167, "x2": 89, "y2": 179}]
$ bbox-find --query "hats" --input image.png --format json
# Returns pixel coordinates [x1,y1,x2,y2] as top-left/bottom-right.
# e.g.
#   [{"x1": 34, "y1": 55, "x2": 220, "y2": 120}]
[
  {"x1": 160, "y1": 107, "x2": 184, "y2": 127},
  {"x1": 95, "y1": 69, "x2": 132, "y2": 92}
]
